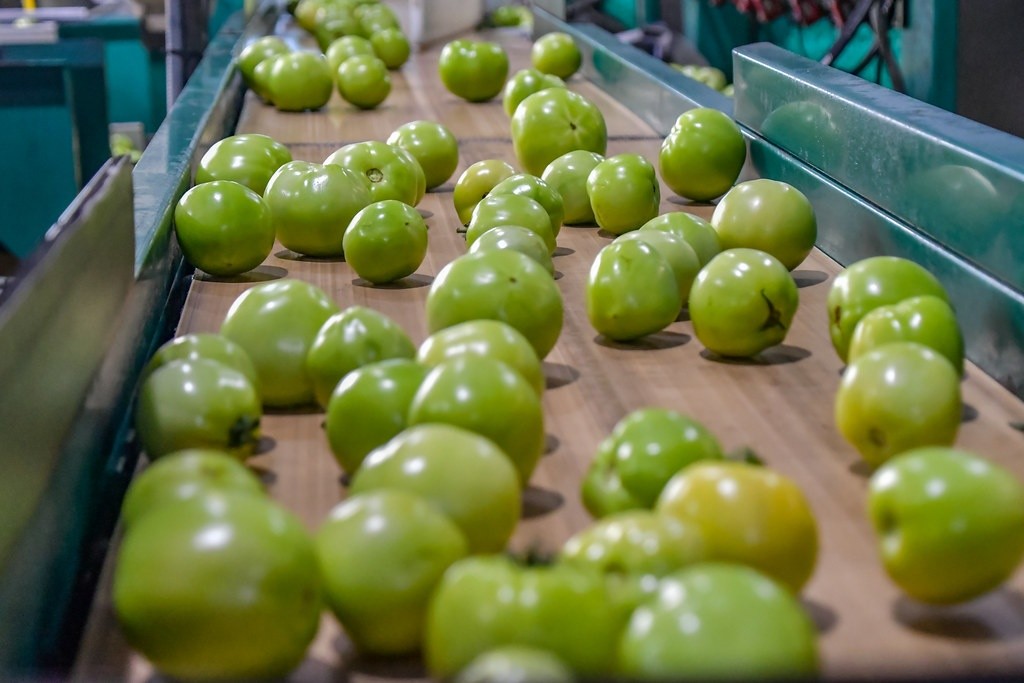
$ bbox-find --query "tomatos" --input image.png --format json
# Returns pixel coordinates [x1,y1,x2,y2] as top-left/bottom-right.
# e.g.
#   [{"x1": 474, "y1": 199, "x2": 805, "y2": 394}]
[{"x1": 110, "y1": 1, "x2": 1024, "y2": 683}]
